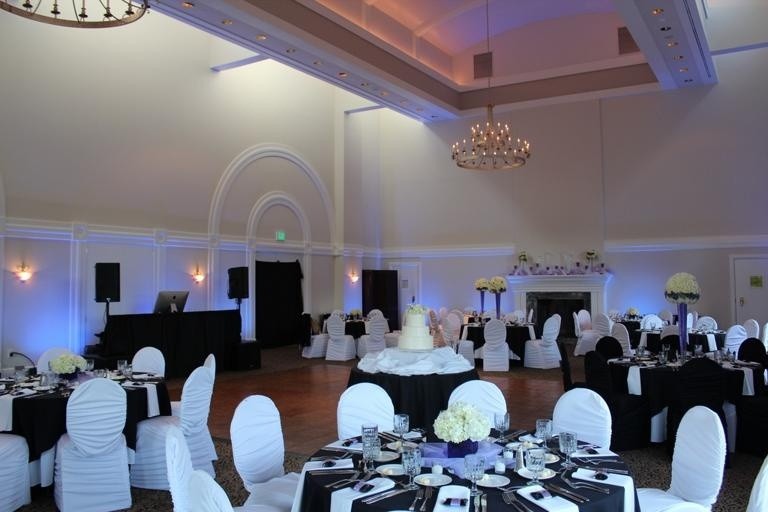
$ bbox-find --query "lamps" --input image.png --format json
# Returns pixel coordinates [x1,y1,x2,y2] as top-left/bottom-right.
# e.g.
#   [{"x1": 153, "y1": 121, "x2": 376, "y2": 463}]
[
  {"x1": 0, "y1": 0, "x2": 150, "y2": 28},
  {"x1": 349, "y1": 268, "x2": 359, "y2": 284},
  {"x1": 451, "y1": 0, "x2": 531, "y2": 170},
  {"x1": 193, "y1": 264, "x2": 204, "y2": 283},
  {"x1": 16, "y1": 264, "x2": 32, "y2": 281}
]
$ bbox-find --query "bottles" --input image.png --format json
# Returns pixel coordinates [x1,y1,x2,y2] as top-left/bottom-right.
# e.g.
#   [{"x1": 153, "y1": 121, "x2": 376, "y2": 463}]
[{"x1": 514, "y1": 444, "x2": 527, "y2": 473}]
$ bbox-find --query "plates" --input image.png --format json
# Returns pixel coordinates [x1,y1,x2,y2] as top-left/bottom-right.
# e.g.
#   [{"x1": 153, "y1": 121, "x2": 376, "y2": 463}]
[
  {"x1": 363, "y1": 451, "x2": 400, "y2": 462},
  {"x1": 506, "y1": 441, "x2": 541, "y2": 453},
  {"x1": 375, "y1": 464, "x2": 422, "y2": 477},
  {"x1": 518, "y1": 466, "x2": 557, "y2": 480},
  {"x1": 387, "y1": 441, "x2": 419, "y2": 450},
  {"x1": 524, "y1": 454, "x2": 561, "y2": 465},
  {"x1": 471, "y1": 474, "x2": 512, "y2": 488},
  {"x1": 414, "y1": 472, "x2": 453, "y2": 487}
]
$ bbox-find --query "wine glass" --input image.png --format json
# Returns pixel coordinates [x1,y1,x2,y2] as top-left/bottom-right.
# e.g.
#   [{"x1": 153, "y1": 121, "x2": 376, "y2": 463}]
[
  {"x1": 464, "y1": 454, "x2": 486, "y2": 496},
  {"x1": 524, "y1": 447, "x2": 545, "y2": 487},
  {"x1": 1, "y1": 357, "x2": 134, "y2": 397},
  {"x1": 362, "y1": 436, "x2": 382, "y2": 475},
  {"x1": 393, "y1": 414, "x2": 411, "y2": 446},
  {"x1": 535, "y1": 419, "x2": 553, "y2": 453},
  {"x1": 631, "y1": 342, "x2": 737, "y2": 372},
  {"x1": 494, "y1": 412, "x2": 511, "y2": 443},
  {"x1": 360, "y1": 423, "x2": 379, "y2": 460},
  {"x1": 400, "y1": 448, "x2": 422, "y2": 491},
  {"x1": 650, "y1": 321, "x2": 719, "y2": 335},
  {"x1": 558, "y1": 431, "x2": 580, "y2": 468}
]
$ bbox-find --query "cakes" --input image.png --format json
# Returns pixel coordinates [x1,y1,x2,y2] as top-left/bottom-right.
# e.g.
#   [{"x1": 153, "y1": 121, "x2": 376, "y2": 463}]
[{"x1": 397, "y1": 310, "x2": 434, "y2": 350}]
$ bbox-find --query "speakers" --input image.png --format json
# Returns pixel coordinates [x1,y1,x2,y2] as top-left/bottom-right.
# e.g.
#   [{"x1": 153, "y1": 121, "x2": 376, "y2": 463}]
[
  {"x1": 229, "y1": 267, "x2": 248, "y2": 299},
  {"x1": 96, "y1": 263, "x2": 120, "y2": 302}
]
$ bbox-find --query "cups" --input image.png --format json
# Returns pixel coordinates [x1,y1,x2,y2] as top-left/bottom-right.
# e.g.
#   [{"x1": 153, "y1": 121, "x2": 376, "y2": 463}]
[
  {"x1": 494, "y1": 459, "x2": 506, "y2": 474},
  {"x1": 503, "y1": 447, "x2": 514, "y2": 459},
  {"x1": 431, "y1": 460, "x2": 443, "y2": 473}
]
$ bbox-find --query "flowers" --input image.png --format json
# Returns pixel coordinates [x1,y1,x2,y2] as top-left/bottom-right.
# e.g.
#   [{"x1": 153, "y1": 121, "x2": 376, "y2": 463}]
[
  {"x1": 488, "y1": 276, "x2": 507, "y2": 294},
  {"x1": 475, "y1": 278, "x2": 489, "y2": 291},
  {"x1": 664, "y1": 271, "x2": 702, "y2": 305}
]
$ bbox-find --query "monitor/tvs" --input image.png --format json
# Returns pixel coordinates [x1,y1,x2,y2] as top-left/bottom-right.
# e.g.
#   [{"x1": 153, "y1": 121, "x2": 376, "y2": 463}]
[{"x1": 153, "y1": 291, "x2": 189, "y2": 312}]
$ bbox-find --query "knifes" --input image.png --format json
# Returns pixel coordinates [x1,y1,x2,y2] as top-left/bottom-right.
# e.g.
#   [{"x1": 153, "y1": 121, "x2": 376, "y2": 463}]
[
  {"x1": 366, "y1": 489, "x2": 408, "y2": 504},
  {"x1": 473, "y1": 493, "x2": 481, "y2": 512},
  {"x1": 360, "y1": 488, "x2": 398, "y2": 502},
  {"x1": 480, "y1": 493, "x2": 488, "y2": 512}
]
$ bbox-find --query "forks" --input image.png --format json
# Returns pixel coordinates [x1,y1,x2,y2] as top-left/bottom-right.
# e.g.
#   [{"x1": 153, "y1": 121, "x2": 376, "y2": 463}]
[
  {"x1": 408, "y1": 489, "x2": 424, "y2": 511},
  {"x1": 494, "y1": 429, "x2": 527, "y2": 443},
  {"x1": 419, "y1": 486, "x2": 433, "y2": 511},
  {"x1": 507, "y1": 492, "x2": 536, "y2": 512},
  {"x1": 308, "y1": 451, "x2": 373, "y2": 488},
  {"x1": 498, "y1": 485, "x2": 524, "y2": 491},
  {"x1": 543, "y1": 457, "x2": 629, "y2": 503},
  {"x1": 502, "y1": 492, "x2": 524, "y2": 512}
]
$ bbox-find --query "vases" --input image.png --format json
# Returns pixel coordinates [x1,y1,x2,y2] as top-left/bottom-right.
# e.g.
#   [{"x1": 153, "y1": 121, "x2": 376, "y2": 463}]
[
  {"x1": 480, "y1": 291, "x2": 485, "y2": 313},
  {"x1": 495, "y1": 293, "x2": 501, "y2": 319}
]
